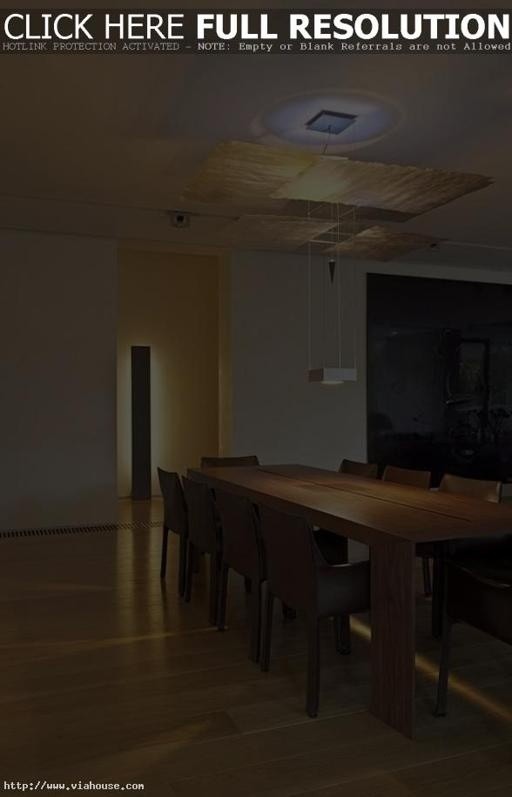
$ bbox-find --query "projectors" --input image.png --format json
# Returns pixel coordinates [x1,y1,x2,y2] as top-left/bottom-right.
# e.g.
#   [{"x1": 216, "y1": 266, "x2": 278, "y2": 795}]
[{"x1": 305, "y1": 367, "x2": 358, "y2": 384}]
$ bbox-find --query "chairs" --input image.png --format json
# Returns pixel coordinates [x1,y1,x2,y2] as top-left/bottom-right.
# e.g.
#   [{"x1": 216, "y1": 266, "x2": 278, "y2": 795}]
[{"x1": 156, "y1": 456, "x2": 504, "y2": 719}]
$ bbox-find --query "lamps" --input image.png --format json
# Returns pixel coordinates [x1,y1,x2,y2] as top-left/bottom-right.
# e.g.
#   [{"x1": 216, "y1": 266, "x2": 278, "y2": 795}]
[{"x1": 304, "y1": 110, "x2": 358, "y2": 383}]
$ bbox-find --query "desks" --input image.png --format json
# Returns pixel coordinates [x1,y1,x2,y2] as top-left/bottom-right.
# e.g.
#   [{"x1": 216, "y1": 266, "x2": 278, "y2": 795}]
[{"x1": 187, "y1": 464, "x2": 511, "y2": 740}]
